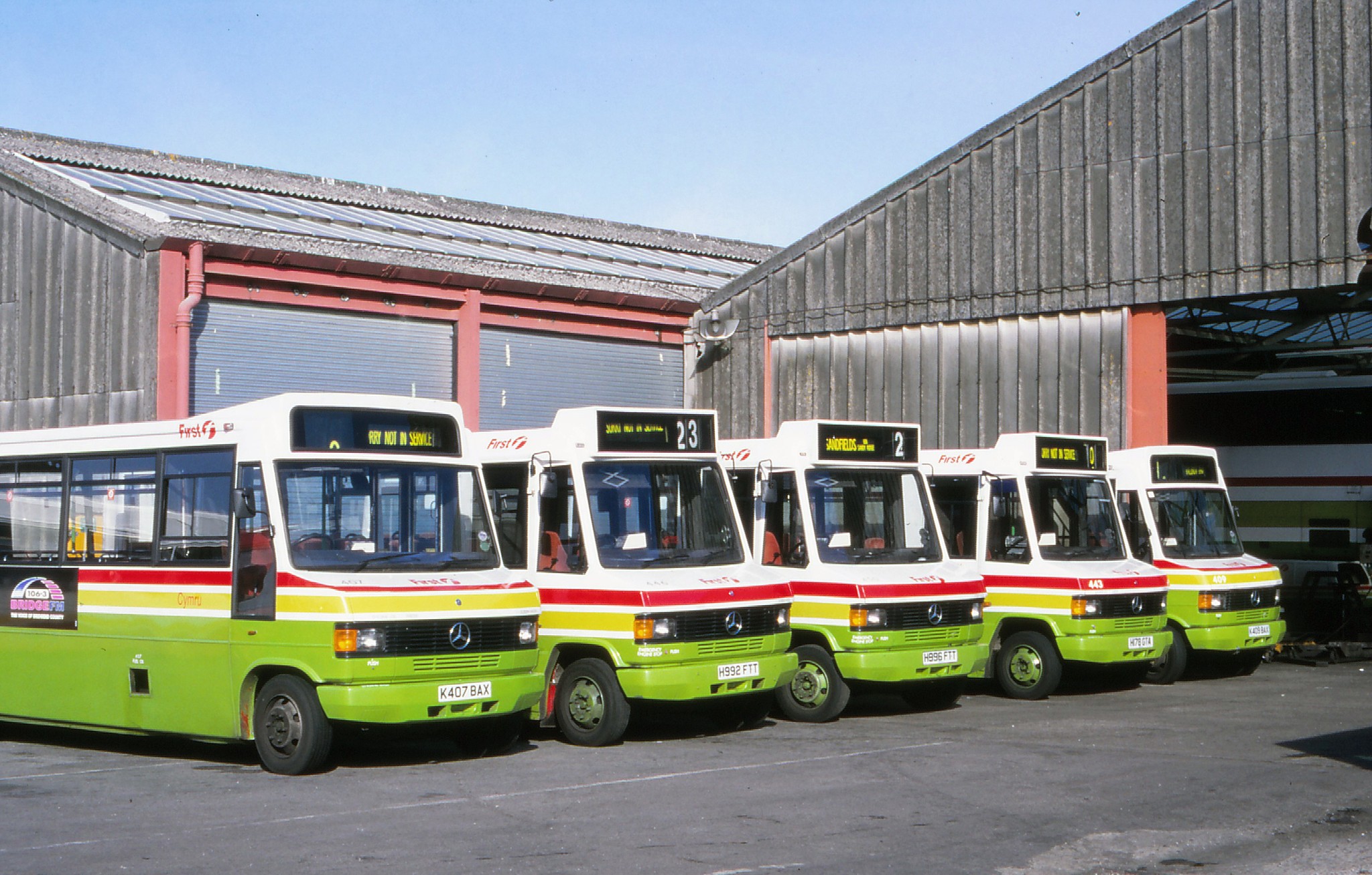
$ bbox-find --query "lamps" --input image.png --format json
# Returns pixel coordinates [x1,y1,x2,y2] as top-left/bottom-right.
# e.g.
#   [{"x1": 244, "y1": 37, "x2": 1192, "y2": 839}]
[{"x1": 695, "y1": 307, "x2": 744, "y2": 342}]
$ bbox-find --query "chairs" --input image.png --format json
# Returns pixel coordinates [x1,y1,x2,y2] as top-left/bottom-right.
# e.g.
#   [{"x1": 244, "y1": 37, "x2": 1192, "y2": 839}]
[
  {"x1": 541, "y1": 530, "x2": 572, "y2": 573},
  {"x1": 762, "y1": 529, "x2": 784, "y2": 565},
  {"x1": 292, "y1": 530, "x2": 338, "y2": 551},
  {"x1": 221, "y1": 508, "x2": 272, "y2": 600},
  {"x1": 862, "y1": 536, "x2": 887, "y2": 549},
  {"x1": 338, "y1": 531, "x2": 366, "y2": 551},
  {"x1": 382, "y1": 530, "x2": 419, "y2": 551},
  {"x1": 955, "y1": 529, "x2": 995, "y2": 561}
]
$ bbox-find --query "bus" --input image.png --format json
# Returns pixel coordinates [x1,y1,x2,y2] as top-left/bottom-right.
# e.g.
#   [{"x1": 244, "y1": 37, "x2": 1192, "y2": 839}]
[
  {"x1": 1164, "y1": 369, "x2": 1372, "y2": 627},
  {"x1": 461, "y1": 405, "x2": 803, "y2": 751},
  {"x1": 1105, "y1": 444, "x2": 1287, "y2": 686},
  {"x1": 0, "y1": 393, "x2": 545, "y2": 777},
  {"x1": 717, "y1": 416, "x2": 988, "y2": 726},
  {"x1": 913, "y1": 432, "x2": 1174, "y2": 710}
]
